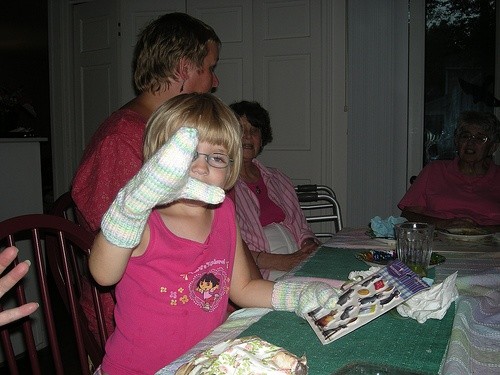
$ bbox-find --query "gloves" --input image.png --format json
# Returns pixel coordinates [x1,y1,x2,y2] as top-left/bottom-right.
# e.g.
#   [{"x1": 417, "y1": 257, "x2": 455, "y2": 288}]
[
  {"x1": 101, "y1": 127, "x2": 225, "y2": 248},
  {"x1": 271, "y1": 281, "x2": 344, "y2": 320}
]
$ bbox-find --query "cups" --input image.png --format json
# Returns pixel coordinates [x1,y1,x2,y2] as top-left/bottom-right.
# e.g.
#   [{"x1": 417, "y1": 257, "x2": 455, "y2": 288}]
[{"x1": 396, "y1": 222, "x2": 435, "y2": 277}]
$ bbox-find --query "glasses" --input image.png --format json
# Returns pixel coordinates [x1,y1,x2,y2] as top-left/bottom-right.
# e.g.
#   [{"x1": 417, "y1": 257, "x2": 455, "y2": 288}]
[{"x1": 458, "y1": 131, "x2": 495, "y2": 146}]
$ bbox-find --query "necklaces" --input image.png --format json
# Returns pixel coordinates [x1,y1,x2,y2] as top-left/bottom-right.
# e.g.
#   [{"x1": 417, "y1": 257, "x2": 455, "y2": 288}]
[{"x1": 255, "y1": 186, "x2": 261, "y2": 193}]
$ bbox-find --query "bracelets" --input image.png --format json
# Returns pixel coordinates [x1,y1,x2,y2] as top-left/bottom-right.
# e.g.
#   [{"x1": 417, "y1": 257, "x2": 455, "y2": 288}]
[
  {"x1": 304, "y1": 240, "x2": 313, "y2": 245},
  {"x1": 256, "y1": 251, "x2": 265, "y2": 263}
]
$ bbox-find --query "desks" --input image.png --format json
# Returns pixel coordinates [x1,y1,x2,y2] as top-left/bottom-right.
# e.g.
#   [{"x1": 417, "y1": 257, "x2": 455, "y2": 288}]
[{"x1": 153, "y1": 229, "x2": 500, "y2": 375}]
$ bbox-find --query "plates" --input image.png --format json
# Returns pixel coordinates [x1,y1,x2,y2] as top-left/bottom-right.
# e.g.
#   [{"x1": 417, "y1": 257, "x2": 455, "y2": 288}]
[
  {"x1": 363, "y1": 250, "x2": 446, "y2": 269},
  {"x1": 438, "y1": 228, "x2": 495, "y2": 241}
]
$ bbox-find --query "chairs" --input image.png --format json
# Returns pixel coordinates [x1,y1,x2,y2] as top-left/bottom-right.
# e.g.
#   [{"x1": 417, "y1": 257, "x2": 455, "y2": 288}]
[
  {"x1": 290, "y1": 185, "x2": 340, "y2": 242},
  {"x1": 43, "y1": 191, "x2": 105, "y2": 362},
  {"x1": 0, "y1": 214, "x2": 115, "y2": 375}
]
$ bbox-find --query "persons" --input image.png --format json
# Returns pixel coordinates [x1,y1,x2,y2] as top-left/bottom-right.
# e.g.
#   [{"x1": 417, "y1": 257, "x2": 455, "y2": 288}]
[
  {"x1": 0, "y1": 246, "x2": 39, "y2": 326},
  {"x1": 70, "y1": 12, "x2": 222, "y2": 344},
  {"x1": 89, "y1": 92, "x2": 341, "y2": 375},
  {"x1": 398, "y1": 111, "x2": 500, "y2": 228},
  {"x1": 228, "y1": 100, "x2": 320, "y2": 282}
]
